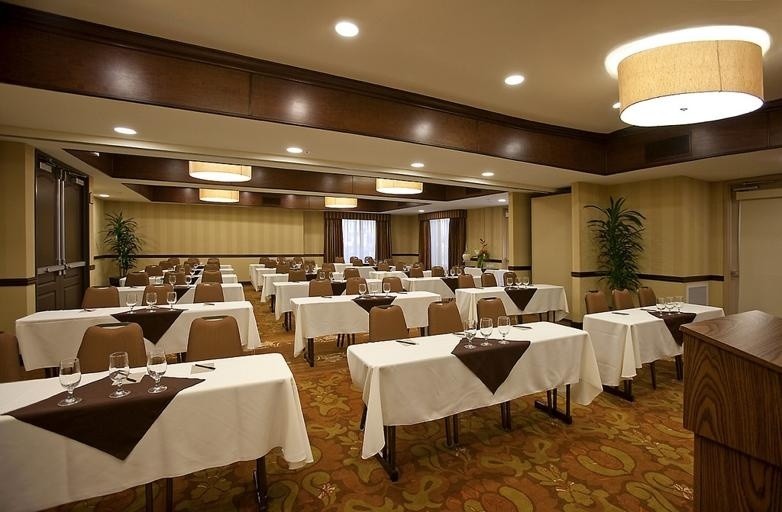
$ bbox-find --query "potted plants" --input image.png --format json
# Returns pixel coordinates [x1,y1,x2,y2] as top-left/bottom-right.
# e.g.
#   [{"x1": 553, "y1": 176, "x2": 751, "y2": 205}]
[{"x1": 96, "y1": 208, "x2": 145, "y2": 286}]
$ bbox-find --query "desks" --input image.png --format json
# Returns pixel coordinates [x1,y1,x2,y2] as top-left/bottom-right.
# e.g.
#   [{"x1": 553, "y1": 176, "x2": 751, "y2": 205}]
[
  {"x1": 2, "y1": 353, "x2": 314, "y2": 510},
  {"x1": 346, "y1": 321, "x2": 604, "y2": 481},
  {"x1": 583, "y1": 302, "x2": 725, "y2": 401}
]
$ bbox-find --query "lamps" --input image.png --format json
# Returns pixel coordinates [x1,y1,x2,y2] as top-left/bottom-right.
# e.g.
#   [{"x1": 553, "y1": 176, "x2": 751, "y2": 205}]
[
  {"x1": 376, "y1": 179, "x2": 424, "y2": 196},
  {"x1": 189, "y1": 161, "x2": 252, "y2": 182},
  {"x1": 605, "y1": 25, "x2": 772, "y2": 128},
  {"x1": 324, "y1": 196, "x2": 358, "y2": 209},
  {"x1": 200, "y1": 188, "x2": 241, "y2": 203}
]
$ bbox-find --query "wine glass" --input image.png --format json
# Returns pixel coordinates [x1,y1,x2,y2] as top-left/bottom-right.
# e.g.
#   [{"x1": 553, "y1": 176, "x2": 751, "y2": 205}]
[
  {"x1": 146, "y1": 351, "x2": 167, "y2": 394},
  {"x1": 656, "y1": 298, "x2": 666, "y2": 318},
  {"x1": 480, "y1": 318, "x2": 493, "y2": 346},
  {"x1": 56, "y1": 357, "x2": 82, "y2": 406},
  {"x1": 108, "y1": 352, "x2": 131, "y2": 399},
  {"x1": 666, "y1": 296, "x2": 678, "y2": 316},
  {"x1": 123, "y1": 258, "x2": 203, "y2": 314},
  {"x1": 498, "y1": 316, "x2": 511, "y2": 345},
  {"x1": 675, "y1": 295, "x2": 687, "y2": 315},
  {"x1": 265, "y1": 256, "x2": 530, "y2": 300},
  {"x1": 464, "y1": 320, "x2": 478, "y2": 349}
]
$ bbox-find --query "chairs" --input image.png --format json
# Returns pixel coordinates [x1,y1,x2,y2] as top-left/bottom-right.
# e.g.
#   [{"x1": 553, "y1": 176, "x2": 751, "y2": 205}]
[
  {"x1": 584, "y1": 290, "x2": 610, "y2": 313},
  {"x1": 611, "y1": 289, "x2": 635, "y2": 310},
  {"x1": 637, "y1": 287, "x2": 659, "y2": 307}
]
